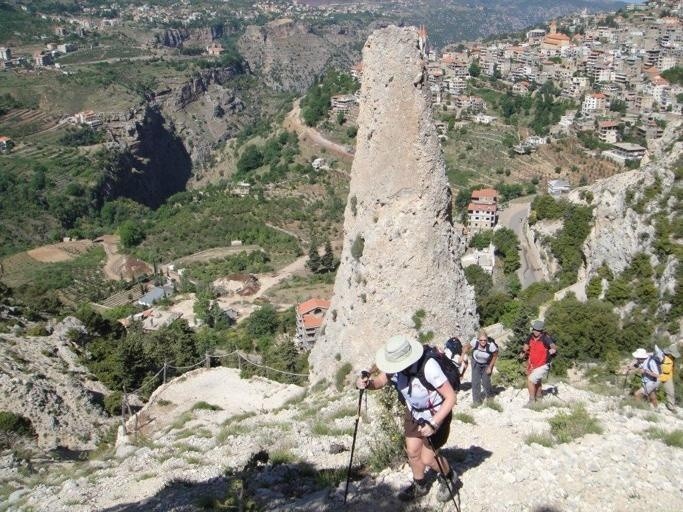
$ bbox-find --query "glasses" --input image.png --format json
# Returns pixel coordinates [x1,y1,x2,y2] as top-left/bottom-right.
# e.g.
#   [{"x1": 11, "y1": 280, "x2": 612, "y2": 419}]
[{"x1": 479, "y1": 339, "x2": 487, "y2": 341}]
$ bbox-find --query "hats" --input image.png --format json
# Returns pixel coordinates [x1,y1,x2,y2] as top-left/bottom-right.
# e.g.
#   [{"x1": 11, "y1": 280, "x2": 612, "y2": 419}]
[
  {"x1": 531, "y1": 320, "x2": 545, "y2": 331},
  {"x1": 374, "y1": 334, "x2": 424, "y2": 374},
  {"x1": 631, "y1": 348, "x2": 650, "y2": 359}
]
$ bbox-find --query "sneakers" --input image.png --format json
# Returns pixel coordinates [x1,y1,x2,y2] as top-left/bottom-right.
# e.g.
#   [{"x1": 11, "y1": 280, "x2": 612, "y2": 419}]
[
  {"x1": 397, "y1": 481, "x2": 428, "y2": 501},
  {"x1": 436, "y1": 466, "x2": 458, "y2": 501}
]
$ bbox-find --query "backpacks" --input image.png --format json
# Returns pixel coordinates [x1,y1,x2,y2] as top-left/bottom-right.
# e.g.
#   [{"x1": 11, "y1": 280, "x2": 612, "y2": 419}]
[
  {"x1": 472, "y1": 337, "x2": 498, "y2": 355},
  {"x1": 645, "y1": 355, "x2": 662, "y2": 376},
  {"x1": 418, "y1": 346, "x2": 462, "y2": 394},
  {"x1": 445, "y1": 337, "x2": 462, "y2": 352}
]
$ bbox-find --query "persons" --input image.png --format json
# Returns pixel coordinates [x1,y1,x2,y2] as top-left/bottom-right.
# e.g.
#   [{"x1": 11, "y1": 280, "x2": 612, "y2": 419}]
[
  {"x1": 355, "y1": 336, "x2": 458, "y2": 503},
  {"x1": 627, "y1": 348, "x2": 660, "y2": 412},
  {"x1": 460, "y1": 331, "x2": 499, "y2": 409},
  {"x1": 437, "y1": 336, "x2": 469, "y2": 376},
  {"x1": 515, "y1": 322, "x2": 556, "y2": 407},
  {"x1": 653, "y1": 344, "x2": 681, "y2": 415}
]
